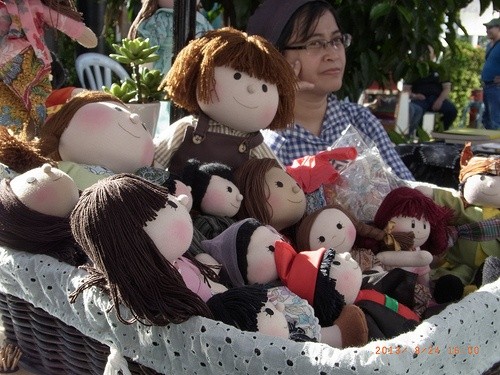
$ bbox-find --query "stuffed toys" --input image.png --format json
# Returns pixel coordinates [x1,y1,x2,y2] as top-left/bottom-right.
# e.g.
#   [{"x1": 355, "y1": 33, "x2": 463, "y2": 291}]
[{"x1": 0, "y1": 0, "x2": 500, "y2": 349}]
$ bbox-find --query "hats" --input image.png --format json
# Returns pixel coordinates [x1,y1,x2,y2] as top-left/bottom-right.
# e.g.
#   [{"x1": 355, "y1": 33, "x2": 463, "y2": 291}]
[
  {"x1": 247, "y1": 0, "x2": 309, "y2": 47},
  {"x1": 483, "y1": 18, "x2": 500, "y2": 26}
]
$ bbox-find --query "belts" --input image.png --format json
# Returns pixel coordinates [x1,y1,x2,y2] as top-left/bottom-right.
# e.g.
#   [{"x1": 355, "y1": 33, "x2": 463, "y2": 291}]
[{"x1": 480, "y1": 80, "x2": 500, "y2": 85}]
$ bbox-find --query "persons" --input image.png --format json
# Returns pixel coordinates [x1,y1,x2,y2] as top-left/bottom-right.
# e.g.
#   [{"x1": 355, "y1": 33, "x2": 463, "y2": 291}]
[
  {"x1": 482, "y1": 18, "x2": 500, "y2": 130},
  {"x1": 402, "y1": 43, "x2": 458, "y2": 146},
  {"x1": 249, "y1": 0, "x2": 416, "y2": 218}
]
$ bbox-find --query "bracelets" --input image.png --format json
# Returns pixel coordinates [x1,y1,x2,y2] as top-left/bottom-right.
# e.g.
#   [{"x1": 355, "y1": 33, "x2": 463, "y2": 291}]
[{"x1": 411, "y1": 94, "x2": 415, "y2": 100}]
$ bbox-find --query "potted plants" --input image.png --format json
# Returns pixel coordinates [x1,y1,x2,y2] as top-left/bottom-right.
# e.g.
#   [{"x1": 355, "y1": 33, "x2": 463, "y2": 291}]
[{"x1": 102, "y1": 35, "x2": 168, "y2": 140}]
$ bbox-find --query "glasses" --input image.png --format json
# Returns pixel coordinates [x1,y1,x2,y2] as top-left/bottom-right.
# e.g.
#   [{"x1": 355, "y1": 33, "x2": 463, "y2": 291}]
[{"x1": 281, "y1": 33, "x2": 351, "y2": 50}]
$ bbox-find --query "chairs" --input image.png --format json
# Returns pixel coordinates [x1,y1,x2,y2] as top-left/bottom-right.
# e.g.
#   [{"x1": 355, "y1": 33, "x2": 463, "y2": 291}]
[{"x1": 75, "y1": 52, "x2": 130, "y2": 92}]
